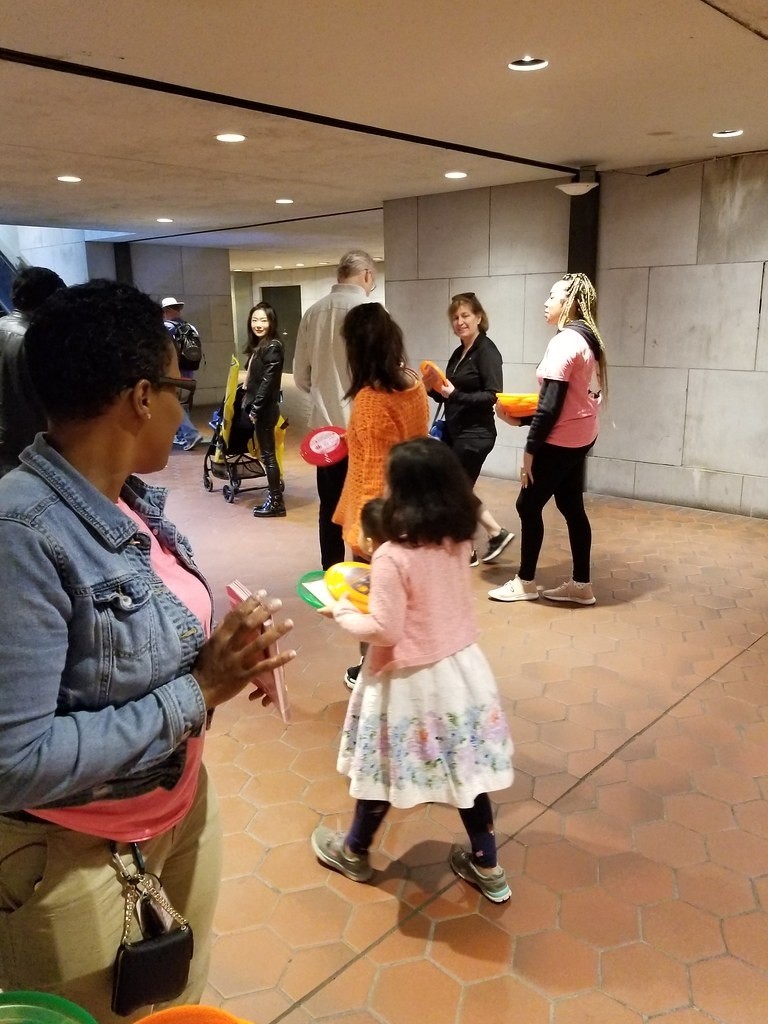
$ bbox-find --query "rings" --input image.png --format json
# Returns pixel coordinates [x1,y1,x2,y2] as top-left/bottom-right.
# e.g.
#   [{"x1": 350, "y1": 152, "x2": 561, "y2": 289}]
[{"x1": 522, "y1": 473, "x2": 526, "y2": 476}]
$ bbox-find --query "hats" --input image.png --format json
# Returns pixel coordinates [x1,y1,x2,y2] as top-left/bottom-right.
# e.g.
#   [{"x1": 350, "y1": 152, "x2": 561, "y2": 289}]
[{"x1": 162, "y1": 297, "x2": 185, "y2": 310}]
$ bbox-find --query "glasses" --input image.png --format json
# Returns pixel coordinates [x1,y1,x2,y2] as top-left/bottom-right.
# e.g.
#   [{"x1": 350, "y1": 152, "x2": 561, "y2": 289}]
[
  {"x1": 452, "y1": 292, "x2": 475, "y2": 300},
  {"x1": 115, "y1": 373, "x2": 197, "y2": 405},
  {"x1": 364, "y1": 269, "x2": 376, "y2": 291}
]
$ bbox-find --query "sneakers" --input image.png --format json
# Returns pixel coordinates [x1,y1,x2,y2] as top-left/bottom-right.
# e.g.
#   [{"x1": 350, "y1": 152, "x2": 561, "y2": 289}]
[
  {"x1": 469, "y1": 549, "x2": 481, "y2": 567},
  {"x1": 345, "y1": 655, "x2": 365, "y2": 689},
  {"x1": 487, "y1": 574, "x2": 540, "y2": 601},
  {"x1": 183, "y1": 434, "x2": 203, "y2": 450},
  {"x1": 484, "y1": 527, "x2": 515, "y2": 561},
  {"x1": 450, "y1": 847, "x2": 512, "y2": 903},
  {"x1": 312, "y1": 824, "x2": 374, "y2": 882},
  {"x1": 542, "y1": 576, "x2": 596, "y2": 604},
  {"x1": 172, "y1": 434, "x2": 187, "y2": 445}
]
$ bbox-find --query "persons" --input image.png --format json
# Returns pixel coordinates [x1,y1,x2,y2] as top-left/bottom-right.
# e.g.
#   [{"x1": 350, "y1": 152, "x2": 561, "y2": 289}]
[
  {"x1": 242, "y1": 303, "x2": 286, "y2": 517},
  {"x1": 161, "y1": 297, "x2": 203, "y2": 450},
  {"x1": 0, "y1": 267, "x2": 67, "y2": 479},
  {"x1": 488, "y1": 273, "x2": 609, "y2": 604},
  {"x1": 422, "y1": 295, "x2": 515, "y2": 567},
  {"x1": 359, "y1": 498, "x2": 385, "y2": 555},
  {"x1": 312, "y1": 437, "x2": 515, "y2": 902},
  {"x1": 291, "y1": 250, "x2": 377, "y2": 570},
  {"x1": 333, "y1": 302, "x2": 430, "y2": 691},
  {"x1": 0, "y1": 265, "x2": 296, "y2": 1024}
]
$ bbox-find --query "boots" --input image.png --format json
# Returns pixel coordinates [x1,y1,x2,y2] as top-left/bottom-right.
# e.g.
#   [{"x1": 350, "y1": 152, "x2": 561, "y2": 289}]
[{"x1": 254, "y1": 492, "x2": 287, "y2": 517}]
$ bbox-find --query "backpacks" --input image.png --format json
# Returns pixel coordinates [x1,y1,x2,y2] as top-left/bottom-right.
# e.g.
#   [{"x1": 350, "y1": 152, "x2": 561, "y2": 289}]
[{"x1": 164, "y1": 318, "x2": 203, "y2": 372}]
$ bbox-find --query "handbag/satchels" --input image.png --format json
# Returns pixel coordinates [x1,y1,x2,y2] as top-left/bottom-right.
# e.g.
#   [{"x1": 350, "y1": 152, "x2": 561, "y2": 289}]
[
  {"x1": 429, "y1": 418, "x2": 447, "y2": 442},
  {"x1": 111, "y1": 876, "x2": 195, "y2": 1017}
]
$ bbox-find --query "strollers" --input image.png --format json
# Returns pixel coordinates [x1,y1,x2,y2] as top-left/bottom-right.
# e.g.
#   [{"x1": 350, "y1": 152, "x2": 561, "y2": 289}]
[{"x1": 203, "y1": 354, "x2": 285, "y2": 504}]
